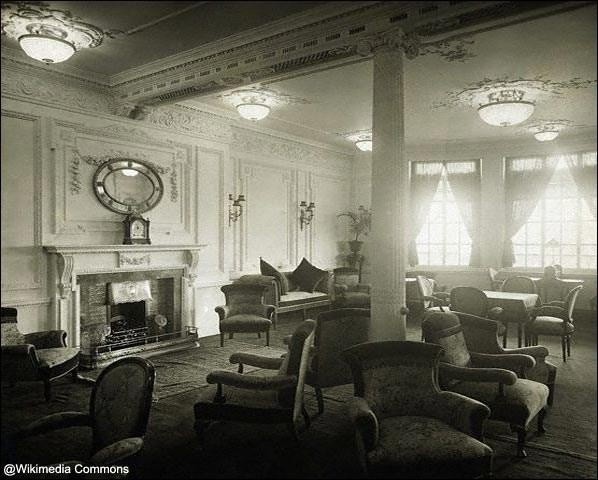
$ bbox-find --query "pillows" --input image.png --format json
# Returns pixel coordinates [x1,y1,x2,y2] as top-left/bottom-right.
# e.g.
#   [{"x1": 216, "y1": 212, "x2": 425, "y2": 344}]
[
  {"x1": 260, "y1": 256, "x2": 289, "y2": 295},
  {"x1": 287, "y1": 257, "x2": 329, "y2": 293}
]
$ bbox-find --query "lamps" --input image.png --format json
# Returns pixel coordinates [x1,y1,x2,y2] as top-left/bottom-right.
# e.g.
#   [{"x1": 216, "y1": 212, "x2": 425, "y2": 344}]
[
  {"x1": 218, "y1": 86, "x2": 311, "y2": 122},
  {"x1": 342, "y1": 128, "x2": 373, "y2": 153},
  {"x1": 300, "y1": 201, "x2": 315, "y2": 230},
  {"x1": 432, "y1": 75, "x2": 597, "y2": 128},
  {"x1": 1, "y1": 1, "x2": 103, "y2": 65},
  {"x1": 512, "y1": 118, "x2": 595, "y2": 142},
  {"x1": 229, "y1": 193, "x2": 245, "y2": 224}
]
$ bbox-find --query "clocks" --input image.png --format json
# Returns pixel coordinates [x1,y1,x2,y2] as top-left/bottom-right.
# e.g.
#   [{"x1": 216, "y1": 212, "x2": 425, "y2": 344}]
[{"x1": 123, "y1": 209, "x2": 152, "y2": 246}]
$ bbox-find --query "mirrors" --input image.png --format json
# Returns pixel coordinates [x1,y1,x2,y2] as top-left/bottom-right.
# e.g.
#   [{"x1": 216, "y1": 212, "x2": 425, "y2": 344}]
[{"x1": 92, "y1": 159, "x2": 164, "y2": 214}]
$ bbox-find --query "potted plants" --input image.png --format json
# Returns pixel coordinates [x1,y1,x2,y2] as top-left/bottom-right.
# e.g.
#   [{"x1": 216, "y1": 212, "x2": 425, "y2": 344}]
[{"x1": 336, "y1": 204, "x2": 371, "y2": 254}]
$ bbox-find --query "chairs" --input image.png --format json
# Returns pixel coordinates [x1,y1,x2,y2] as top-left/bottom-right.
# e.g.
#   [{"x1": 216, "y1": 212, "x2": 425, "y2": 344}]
[
  {"x1": 1, "y1": 307, "x2": 98, "y2": 411},
  {"x1": 190, "y1": 268, "x2": 584, "y2": 480},
  {"x1": 23, "y1": 357, "x2": 156, "y2": 479}
]
